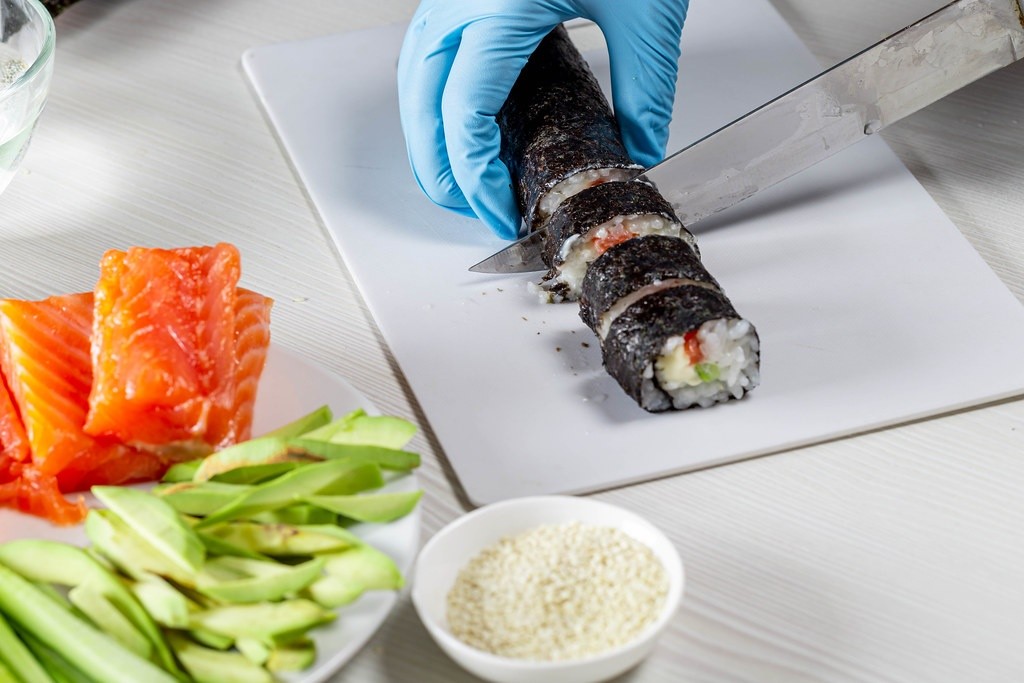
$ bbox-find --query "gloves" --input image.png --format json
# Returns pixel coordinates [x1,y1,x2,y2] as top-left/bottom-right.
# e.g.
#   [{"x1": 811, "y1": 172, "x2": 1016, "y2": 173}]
[{"x1": 397, "y1": 1, "x2": 688, "y2": 242}]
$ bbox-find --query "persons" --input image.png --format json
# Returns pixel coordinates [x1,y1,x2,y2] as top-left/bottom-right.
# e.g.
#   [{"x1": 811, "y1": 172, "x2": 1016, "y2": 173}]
[{"x1": 396, "y1": 0, "x2": 690, "y2": 241}]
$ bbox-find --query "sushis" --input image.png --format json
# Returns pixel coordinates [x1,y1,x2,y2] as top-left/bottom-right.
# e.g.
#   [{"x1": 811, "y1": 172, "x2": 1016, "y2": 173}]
[{"x1": 457, "y1": 7, "x2": 762, "y2": 411}]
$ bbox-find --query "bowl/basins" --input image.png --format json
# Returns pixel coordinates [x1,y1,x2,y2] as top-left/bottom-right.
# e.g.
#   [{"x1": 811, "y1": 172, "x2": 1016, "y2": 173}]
[
  {"x1": 412, "y1": 495, "x2": 686, "y2": 683},
  {"x1": 0, "y1": 0, "x2": 56, "y2": 196}
]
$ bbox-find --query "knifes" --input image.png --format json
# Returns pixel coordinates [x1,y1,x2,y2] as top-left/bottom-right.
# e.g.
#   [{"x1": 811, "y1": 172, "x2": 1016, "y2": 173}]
[{"x1": 468, "y1": 0, "x2": 1024, "y2": 273}]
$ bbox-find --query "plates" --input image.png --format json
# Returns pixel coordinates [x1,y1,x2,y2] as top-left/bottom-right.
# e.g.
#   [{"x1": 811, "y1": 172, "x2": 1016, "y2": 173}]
[{"x1": 0, "y1": 341, "x2": 424, "y2": 683}]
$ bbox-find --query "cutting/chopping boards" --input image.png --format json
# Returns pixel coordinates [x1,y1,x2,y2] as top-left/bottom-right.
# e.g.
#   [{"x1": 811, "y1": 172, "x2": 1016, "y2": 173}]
[{"x1": 241, "y1": 0, "x2": 1024, "y2": 506}]
